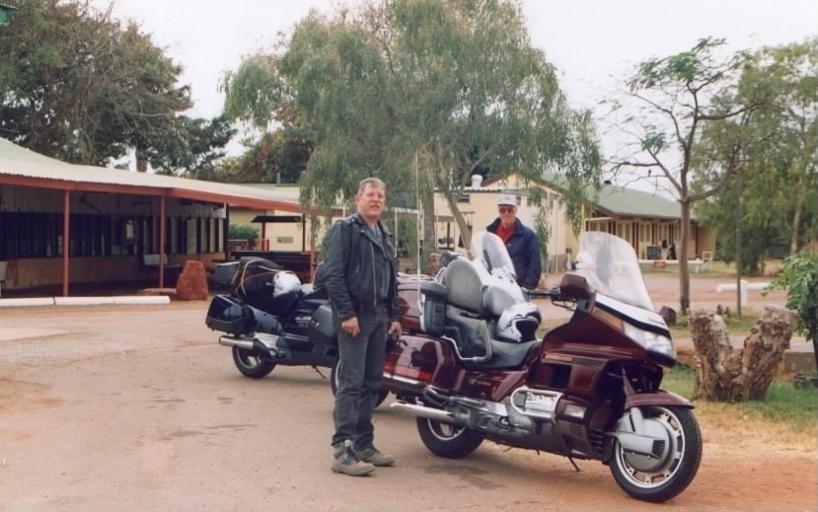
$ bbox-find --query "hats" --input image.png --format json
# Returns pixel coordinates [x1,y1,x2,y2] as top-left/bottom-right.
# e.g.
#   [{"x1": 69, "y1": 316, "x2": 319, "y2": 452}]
[{"x1": 497, "y1": 195, "x2": 518, "y2": 206}]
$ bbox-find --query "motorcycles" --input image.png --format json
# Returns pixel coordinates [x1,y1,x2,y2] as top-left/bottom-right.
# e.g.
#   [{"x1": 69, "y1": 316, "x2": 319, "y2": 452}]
[{"x1": 205, "y1": 256, "x2": 390, "y2": 409}]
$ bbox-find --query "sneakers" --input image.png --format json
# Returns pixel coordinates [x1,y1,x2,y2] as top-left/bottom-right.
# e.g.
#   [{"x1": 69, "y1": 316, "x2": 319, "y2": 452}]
[{"x1": 331, "y1": 439, "x2": 395, "y2": 476}]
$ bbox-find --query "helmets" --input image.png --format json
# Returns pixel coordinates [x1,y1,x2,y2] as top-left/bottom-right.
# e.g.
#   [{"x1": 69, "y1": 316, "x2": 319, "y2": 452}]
[
  {"x1": 496, "y1": 302, "x2": 543, "y2": 343},
  {"x1": 271, "y1": 270, "x2": 301, "y2": 298}
]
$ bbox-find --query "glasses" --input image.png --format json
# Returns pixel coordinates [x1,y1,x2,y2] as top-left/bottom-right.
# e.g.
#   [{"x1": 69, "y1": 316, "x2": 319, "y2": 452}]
[{"x1": 499, "y1": 208, "x2": 514, "y2": 214}]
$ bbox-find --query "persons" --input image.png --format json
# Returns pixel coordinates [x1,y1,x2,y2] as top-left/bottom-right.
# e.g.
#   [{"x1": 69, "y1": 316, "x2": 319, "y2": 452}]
[
  {"x1": 484, "y1": 194, "x2": 541, "y2": 303},
  {"x1": 319, "y1": 176, "x2": 403, "y2": 478}
]
